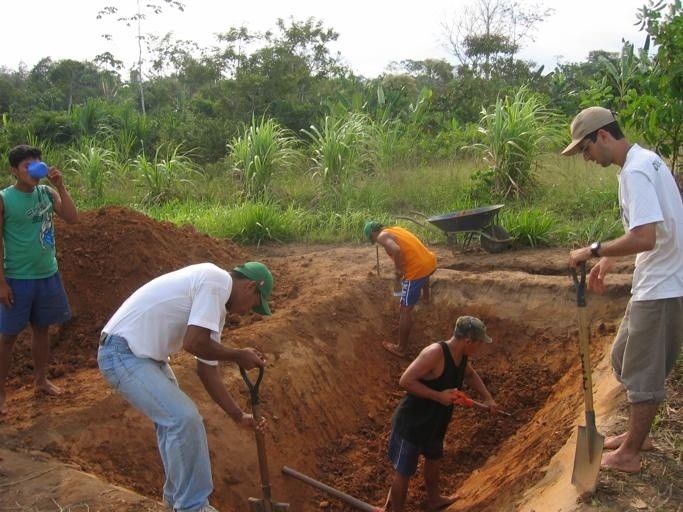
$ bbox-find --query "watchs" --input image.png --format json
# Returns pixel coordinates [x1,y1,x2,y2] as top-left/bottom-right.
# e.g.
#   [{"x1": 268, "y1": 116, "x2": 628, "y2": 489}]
[{"x1": 590, "y1": 240, "x2": 600, "y2": 258}]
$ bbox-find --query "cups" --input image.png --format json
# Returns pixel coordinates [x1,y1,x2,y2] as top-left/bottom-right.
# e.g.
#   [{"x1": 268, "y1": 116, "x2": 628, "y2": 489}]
[{"x1": 27, "y1": 162, "x2": 50, "y2": 179}]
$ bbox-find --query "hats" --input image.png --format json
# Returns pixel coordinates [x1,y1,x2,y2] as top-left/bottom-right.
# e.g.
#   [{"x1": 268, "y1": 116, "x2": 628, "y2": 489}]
[
  {"x1": 234, "y1": 262, "x2": 273, "y2": 315},
  {"x1": 561, "y1": 106, "x2": 616, "y2": 155},
  {"x1": 364, "y1": 221, "x2": 379, "y2": 244},
  {"x1": 455, "y1": 316, "x2": 492, "y2": 344}
]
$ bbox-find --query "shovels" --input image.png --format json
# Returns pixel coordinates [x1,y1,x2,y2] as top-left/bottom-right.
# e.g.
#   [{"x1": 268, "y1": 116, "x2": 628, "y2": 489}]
[
  {"x1": 451, "y1": 390, "x2": 537, "y2": 420},
  {"x1": 570, "y1": 262, "x2": 606, "y2": 493},
  {"x1": 239, "y1": 356, "x2": 290, "y2": 512}
]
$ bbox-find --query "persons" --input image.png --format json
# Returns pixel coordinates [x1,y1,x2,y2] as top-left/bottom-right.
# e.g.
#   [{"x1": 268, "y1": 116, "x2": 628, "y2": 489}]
[
  {"x1": 97, "y1": 262, "x2": 273, "y2": 512},
  {"x1": 561, "y1": 107, "x2": 683, "y2": 476},
  {"x1": 385, "y1": 316, "x2": 497, "y2": 512},
  {"x1": 364, "y1": 222, "x2": 438, "y2": 358},
  {"x1": 0, "y1": 145, "x2": 80, "y2": 415}
]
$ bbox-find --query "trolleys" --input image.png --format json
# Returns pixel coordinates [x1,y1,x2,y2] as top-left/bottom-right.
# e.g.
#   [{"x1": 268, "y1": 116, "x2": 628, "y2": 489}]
[{"x1": 392, "y1": 202, "x2": 522, "y2": 256}]
